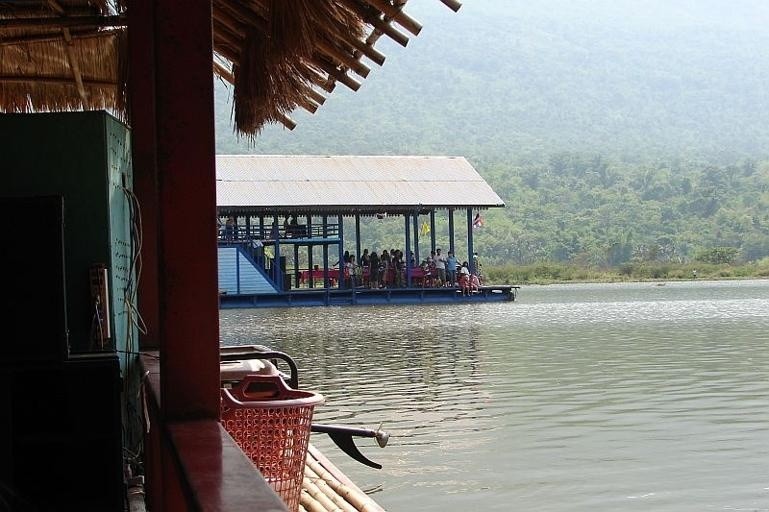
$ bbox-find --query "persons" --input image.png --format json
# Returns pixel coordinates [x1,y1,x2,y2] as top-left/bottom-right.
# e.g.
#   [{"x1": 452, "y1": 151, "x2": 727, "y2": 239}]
[
  {"x1": 333, "y1": 248, "x2": 482, "y2": 297},
  {"x1": 225, "y1": 214, "x2": 236, "y2": 247},
  {"x1": 283, "y1": 215, "x2": 289, "y2": 239},
  {"x1": 290, "y1": 216, "x2": 298, "y2": 239}
]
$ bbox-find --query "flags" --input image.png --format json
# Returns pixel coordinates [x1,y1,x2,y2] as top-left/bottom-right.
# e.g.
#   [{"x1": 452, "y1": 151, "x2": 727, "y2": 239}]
[
  {"x1": 420, "y1": 220, "x2": 430, "y2": 236},
  {"x1": 473, "y1": 213, "x2": 483, "y2": 228}
]
